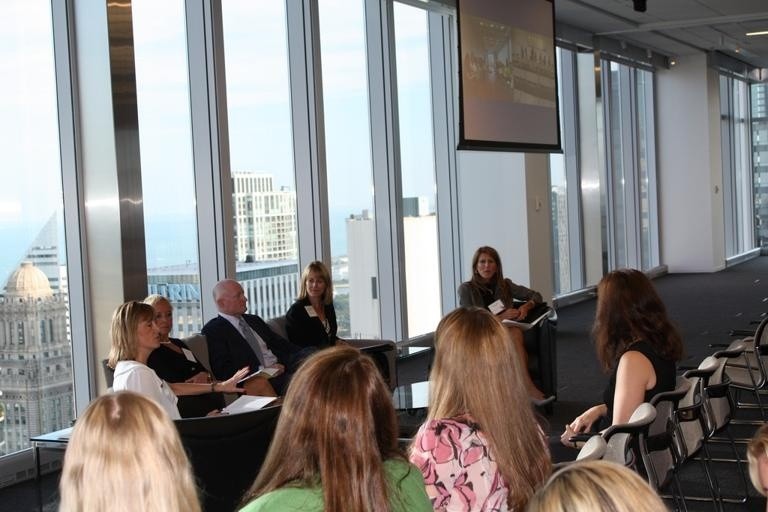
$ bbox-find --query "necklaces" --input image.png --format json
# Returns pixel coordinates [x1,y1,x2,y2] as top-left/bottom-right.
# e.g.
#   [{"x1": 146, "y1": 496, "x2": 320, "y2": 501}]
[{"x1": 159, "y1": 339, "x2": 171, "y2": 343}]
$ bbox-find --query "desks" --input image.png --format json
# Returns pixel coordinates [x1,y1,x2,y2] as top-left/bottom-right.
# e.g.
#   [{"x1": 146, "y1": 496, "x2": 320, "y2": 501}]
[
  {"x1": 397, "y1": 346, "x2": 433, "y2": 418},
  {"x1": 30, "y1": 426, "x2": 73, "y2": 512}
]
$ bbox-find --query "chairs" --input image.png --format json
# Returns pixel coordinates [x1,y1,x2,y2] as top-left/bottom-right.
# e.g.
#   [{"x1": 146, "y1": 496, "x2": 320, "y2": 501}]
[
  {"x1": 512, "y1": 303, "x2": 557, "y2": 406},
  {"x1": 267, "y1": 316, "x2": 398, "y2": 392},
  {"x1": 183, "y1": 334, "x2": 211, "y2": 370},
  {"x1": 174, "y1": 404, "x2": 283, "y2": 512}
]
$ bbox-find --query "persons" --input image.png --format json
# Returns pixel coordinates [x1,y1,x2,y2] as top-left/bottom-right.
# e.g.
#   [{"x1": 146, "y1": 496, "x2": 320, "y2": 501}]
[
  {"x1": 746, "y1": 422, "x2": 768, "y2": 512},
  {"x1": 401, "y1": 305, "x2": 550, "y2": 512},
  {"x1": 105, "y1": 301, "x2": 250, "y2": 419},
  {"x1": 143, "y1": 294, "x2": 250, "y2": 418},
  {"x1": 236, "y1": 347, "x2": 433, "y2": 512},
  {"x1": 57, "y1": 390, "x2": 202, "y2": 512},
  {"x1": 559, "y1": 268, "x2": 684, "y2": 450},
  {"x1": 458, "y1": 246, "x2": 556, "y2": 406},
  {"x1": 527, "y1": 459, "x2": 671, "y2": 512},
  {"x1": 285, "y1": 261, "x2": 351, "y2": 360},
  {"x1": 201, "y1": 279, "x2": 304, "y2": 396}
]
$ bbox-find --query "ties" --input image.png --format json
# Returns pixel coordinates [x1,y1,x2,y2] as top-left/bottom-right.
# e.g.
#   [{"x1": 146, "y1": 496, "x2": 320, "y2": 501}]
[{"x1": 239, "y1": 319, "x2": 264, "y2": 365}]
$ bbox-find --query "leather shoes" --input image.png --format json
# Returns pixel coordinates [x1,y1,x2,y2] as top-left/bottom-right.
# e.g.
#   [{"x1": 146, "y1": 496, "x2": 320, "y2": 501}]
[{"x1": 530, "y1": 393, "x2": 556, "y2": 407}]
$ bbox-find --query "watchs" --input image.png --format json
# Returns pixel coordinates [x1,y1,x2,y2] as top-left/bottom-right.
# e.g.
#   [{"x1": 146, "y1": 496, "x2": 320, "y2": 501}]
[{"x1": 211, "y1": 381, "x2": 221, "y2": 392}]
